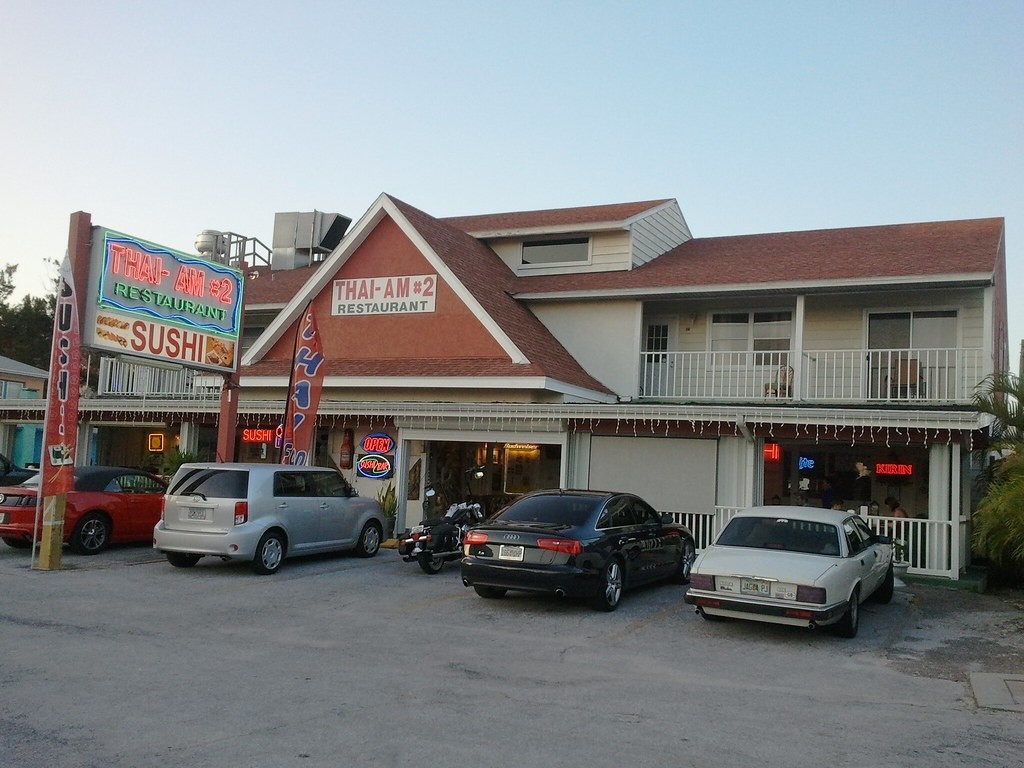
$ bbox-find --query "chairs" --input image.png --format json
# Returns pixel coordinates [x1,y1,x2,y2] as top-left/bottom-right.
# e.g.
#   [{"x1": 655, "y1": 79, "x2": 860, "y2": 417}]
[
  {"x1": 885, "y1": 359, "x2": 926, "y2": 402},
  {"x1": 764, "y1": 365, "x2": 794, "y2": 401}
]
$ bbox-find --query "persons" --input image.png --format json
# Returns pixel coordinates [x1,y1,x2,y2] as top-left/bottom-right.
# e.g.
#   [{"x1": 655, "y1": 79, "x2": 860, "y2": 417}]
[
  {"x1": 772, "y1": 494, "x2": 781, "y2": 505},
  {"x1": 884, "y1": 497, "x2": 908, "y2": 542},
  {"x1": 870, "y1": 500, "x2": 880, "y2": 516},
  {"x1": 830, "y1": 499, "x2": 844, "y2": 511},
  {"x1": 142, "y1": 459, "x2": 157, "y2": 474},
  {"x1": 795, "y1": 493, "x2": 809, "y2": 506},
  {"x1": 857, "y1": 504, "x2": 869, "y2": 515}
]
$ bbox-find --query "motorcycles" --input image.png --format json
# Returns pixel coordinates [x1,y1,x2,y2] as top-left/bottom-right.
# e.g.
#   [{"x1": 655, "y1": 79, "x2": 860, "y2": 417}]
[{"x1": 396, "y1": 488, "x2": 487, "y2": 575}]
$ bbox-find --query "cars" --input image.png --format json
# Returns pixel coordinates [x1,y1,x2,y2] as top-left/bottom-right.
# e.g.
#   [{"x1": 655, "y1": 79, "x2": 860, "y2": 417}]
[
  {"x1": 0, "y1": 465, "x2": 169, "y2": 555},
  {"x1": 682, "y1": 503, "x2": 899, "y2": 640},
  {"x1": 0, "y1": 453, "x2": 41, "y2": 487},
  {"x1": 461, "y1": 488, "x2": 694, "y2": 612},
  {"x1": 149, "y1": 463, "x2": 389, "y2": 576}
]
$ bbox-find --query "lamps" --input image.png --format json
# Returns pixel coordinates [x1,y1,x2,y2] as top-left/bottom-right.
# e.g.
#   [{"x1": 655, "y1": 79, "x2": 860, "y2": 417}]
[
  {"x1": 248, "y1": 269, "x2": 259, "y2": 280},
  {"x1": 616, "y1": 394, "x2": 633, "y2": 403}
]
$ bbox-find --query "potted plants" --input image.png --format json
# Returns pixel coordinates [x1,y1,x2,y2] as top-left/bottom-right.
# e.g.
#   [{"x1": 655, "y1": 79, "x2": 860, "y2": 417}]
[
  {"x1": 376, "y1": 481, "x2": 399, "y2": 539},
  {"x1": 892, "y1": 538, "x2": 911, "y2": 587}
]
